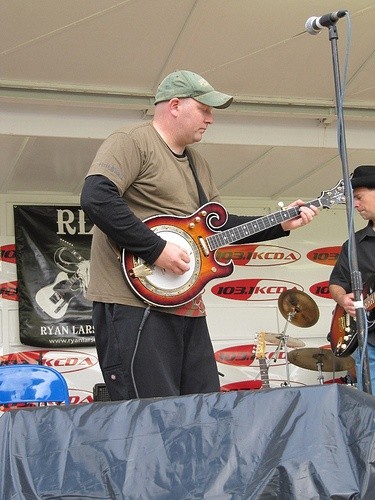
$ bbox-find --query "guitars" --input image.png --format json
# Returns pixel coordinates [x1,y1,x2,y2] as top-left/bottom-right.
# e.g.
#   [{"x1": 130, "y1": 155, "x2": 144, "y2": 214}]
[
  {"x1": 329, "y1": 292, "x2": 375, "y2": 360},
  {"x1": 252, "y1": 331, "x2": 271, "y2": 389},
  {"x1": 116, "y1": 179, "x2": 354, "y2": 311},
  {"x1": 33, "y1": 274, "x2": 78, "y2": 321}
]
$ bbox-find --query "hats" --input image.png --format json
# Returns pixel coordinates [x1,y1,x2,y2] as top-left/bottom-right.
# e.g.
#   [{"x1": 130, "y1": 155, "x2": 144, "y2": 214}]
[
  {"x1": 351, "y1": 165, "x2": 375, "y2": 188},
  {"x1": 154, "y1": 71, "x2": 233, "y2": 109}
]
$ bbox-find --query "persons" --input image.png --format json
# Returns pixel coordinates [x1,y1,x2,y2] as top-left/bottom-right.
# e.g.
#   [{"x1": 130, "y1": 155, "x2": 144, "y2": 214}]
[
  {"x1": 329, "y1": 166, "x2": 375, "y2": 395},
  {"x1": 80, "y1": 70, "x2": 319, "y2": 402}
]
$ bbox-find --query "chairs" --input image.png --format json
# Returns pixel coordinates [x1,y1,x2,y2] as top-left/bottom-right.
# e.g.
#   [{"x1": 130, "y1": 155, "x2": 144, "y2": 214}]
[{"x1": 0, "y1": 364, "x2": 70, "y2": 406}]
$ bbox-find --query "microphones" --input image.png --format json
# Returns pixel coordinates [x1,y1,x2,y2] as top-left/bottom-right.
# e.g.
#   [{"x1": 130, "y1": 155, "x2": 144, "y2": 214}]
[{"x1": 304, "y1": 9, "x2": 348, "y2": 35}]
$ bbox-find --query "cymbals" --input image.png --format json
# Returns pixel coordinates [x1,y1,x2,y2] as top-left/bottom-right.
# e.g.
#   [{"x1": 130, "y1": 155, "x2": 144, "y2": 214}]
[
  {"x1": 288, "y1": 346, "x2": 355, "y2": 374},
  {"x1": 274, "y1": 288, "x2": 320, "y2": 330},
  {"x1": 263, "y1": 331, "x2": 305, "y2": 349}
]
